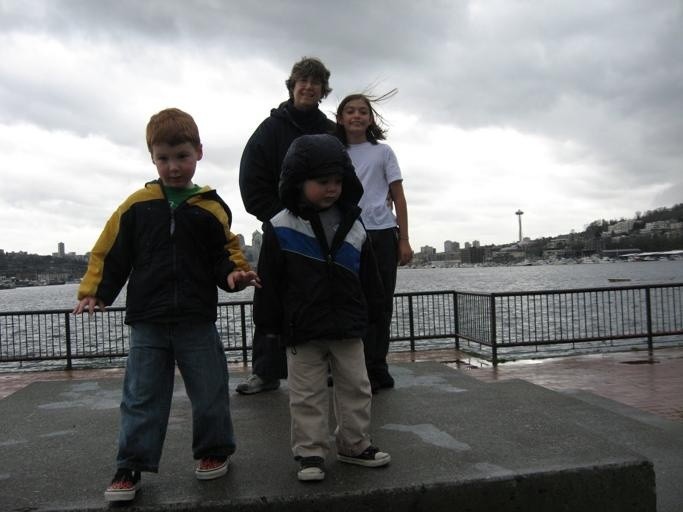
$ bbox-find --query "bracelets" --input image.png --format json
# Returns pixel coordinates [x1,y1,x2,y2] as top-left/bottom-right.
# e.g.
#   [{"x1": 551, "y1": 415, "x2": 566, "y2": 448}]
[{"x1": 399, "y1": 235, "x2": 409, "y2": 240}]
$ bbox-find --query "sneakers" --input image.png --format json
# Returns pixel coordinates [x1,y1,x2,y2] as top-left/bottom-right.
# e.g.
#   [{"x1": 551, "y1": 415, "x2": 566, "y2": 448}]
[
  {"x1": 295, "y1": 454, "x2": 325, "y2": 481},
  {"x1": 336, "y1": 444, "x2": 391, "y2": 468},
  {"x1": 236, "y1": 373, "x2": 281, "y2": 395},
  {"x1": 195, "y1": 454, "x2": 232, "y2": 480},
  {"x1": 379, "y1": 366, "x2": 394, "y2": 389},
  {"x1": 103, "y1": 467, "x2": 143, "y2": 502}
]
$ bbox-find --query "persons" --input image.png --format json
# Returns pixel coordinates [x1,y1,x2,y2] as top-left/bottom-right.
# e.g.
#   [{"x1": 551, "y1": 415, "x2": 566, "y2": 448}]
[
  {"x1": 237, "y1": 56, "x2": 343, "y2": 397},
  {"x1": 326, "y1": 93, "x2": 413, "y2": 397},
  {"x1": 71, "y1": 107, "x2": 262, "y2": 502},
  {"x1": 250, "y1": 132, "x2": 390, "y2": 480}
]
